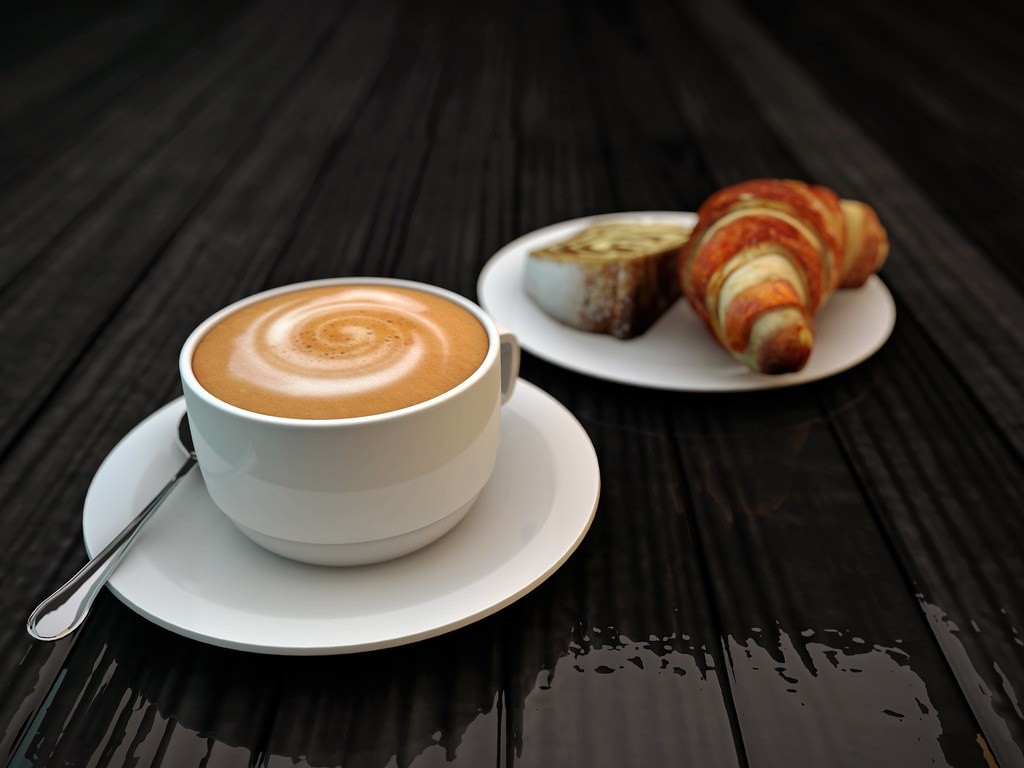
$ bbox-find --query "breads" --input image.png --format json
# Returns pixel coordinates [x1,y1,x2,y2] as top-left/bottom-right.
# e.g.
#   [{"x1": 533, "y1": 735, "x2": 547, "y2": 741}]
[
  {"x1": 680, "y1": 180, "x2": 889, "y2": 375},
  {"x1": 524, "y1": 222, "x2": 688, "y2": 341}
]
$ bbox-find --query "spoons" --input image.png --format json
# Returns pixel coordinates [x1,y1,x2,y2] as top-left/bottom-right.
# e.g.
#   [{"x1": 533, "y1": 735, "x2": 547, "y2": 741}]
[{"x1": 27, "y1": 412, "x2": 199, "y2": 641}]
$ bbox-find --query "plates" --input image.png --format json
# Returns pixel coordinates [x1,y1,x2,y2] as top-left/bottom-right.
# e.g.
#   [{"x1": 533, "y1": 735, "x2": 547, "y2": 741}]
[
  {"x1": 83, "y1": 376, "x2": 600, "y2": 655},
  {"x1": 477, "y1": 212, "x2": 896, "y2": 392}
]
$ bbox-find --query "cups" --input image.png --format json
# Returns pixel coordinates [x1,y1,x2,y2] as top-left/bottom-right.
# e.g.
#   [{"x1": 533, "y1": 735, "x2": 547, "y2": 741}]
[{"x1": 179, "y1": 277, "x2": 519, "y2": 567}]
